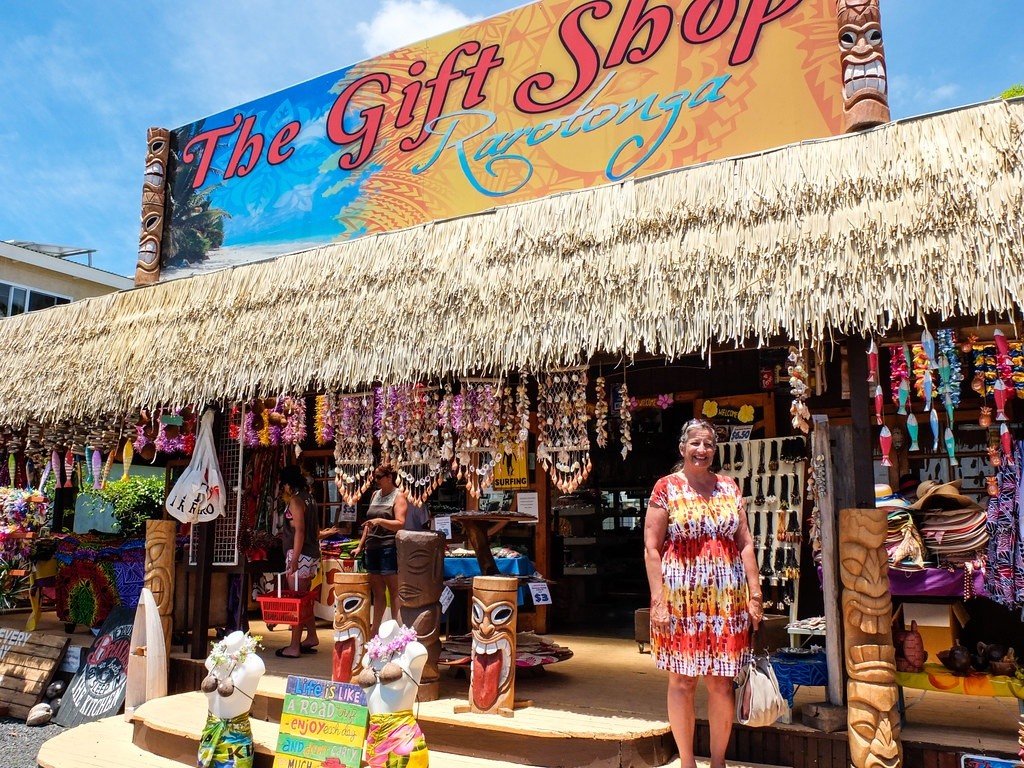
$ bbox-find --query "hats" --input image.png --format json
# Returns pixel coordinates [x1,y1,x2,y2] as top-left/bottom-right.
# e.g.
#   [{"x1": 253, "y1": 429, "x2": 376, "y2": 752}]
[{"x1": 874, "y1": 478, "x2": 990, "y2": 573}]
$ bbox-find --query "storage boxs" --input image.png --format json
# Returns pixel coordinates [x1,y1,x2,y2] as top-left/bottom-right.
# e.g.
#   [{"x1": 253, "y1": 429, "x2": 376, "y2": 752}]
[{"x1": 892, "y1": 599, "x2": 971, "y2": 664}]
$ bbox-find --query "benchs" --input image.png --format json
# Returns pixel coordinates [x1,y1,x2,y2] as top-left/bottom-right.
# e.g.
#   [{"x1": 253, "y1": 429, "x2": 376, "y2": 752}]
[{"x1": 634, "y1": 607, "x2": 789, "y2": 657}]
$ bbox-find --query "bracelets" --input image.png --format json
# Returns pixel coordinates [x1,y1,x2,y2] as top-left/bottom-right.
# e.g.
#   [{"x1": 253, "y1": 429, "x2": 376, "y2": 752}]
[{"x1": 751, "y1": 593, "x2": 763, "y2": 599}]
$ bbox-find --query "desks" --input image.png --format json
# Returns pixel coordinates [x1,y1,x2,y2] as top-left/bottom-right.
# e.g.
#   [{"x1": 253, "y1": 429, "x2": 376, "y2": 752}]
[
  {"x1": 446, "y1": 514, "x2": 538, "y2": 577},
  {"x1": 441, "y1": 556, "x2": 534, "y2": 631},
  {"x1": 55, "y1": 535, "x2": 145, "y2": 634},
  {"x1": 817, "y1": 568, "x2": 986, "y2": 594},
  {"x1": 314, "y1": 559, "x2": 363, "y2": 621}
]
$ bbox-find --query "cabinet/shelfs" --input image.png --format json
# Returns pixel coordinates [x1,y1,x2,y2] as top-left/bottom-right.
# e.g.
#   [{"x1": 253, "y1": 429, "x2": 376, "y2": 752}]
[{"x1": 561, "y1": 506, "x2": 645, "y2": 576}]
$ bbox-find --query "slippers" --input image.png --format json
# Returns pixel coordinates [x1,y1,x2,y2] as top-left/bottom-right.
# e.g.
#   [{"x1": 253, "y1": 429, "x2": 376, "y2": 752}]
[{"x1": 275, "y1": 641, "x2": 320, "y2": 659}]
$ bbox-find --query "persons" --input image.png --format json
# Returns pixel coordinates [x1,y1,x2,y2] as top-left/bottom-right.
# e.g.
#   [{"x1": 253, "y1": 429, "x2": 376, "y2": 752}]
[
  {"x1": 197, "y1": 630, "x2": 266, "y2": 768},
  {"x1": 643, "y1": 418, "x2": 764, "y2": 768},
  {"x1": 404, "y1": 486, "x2": 431, "y2": 531},
  {"x1": 275, "y1": 465, "x2": 321, "y2": 658},
  {"x1": 357, "y1": 620, "x2": 429, "y2": 768},
  {"x1": 350, "y1": 463, "x2": 408, "y2": 641}
]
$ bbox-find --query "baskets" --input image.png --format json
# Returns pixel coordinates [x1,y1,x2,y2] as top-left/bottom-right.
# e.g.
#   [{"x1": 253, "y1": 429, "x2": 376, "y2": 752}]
[{"x1": 257, "y1": 569, "x2": 316, "y2": 626}]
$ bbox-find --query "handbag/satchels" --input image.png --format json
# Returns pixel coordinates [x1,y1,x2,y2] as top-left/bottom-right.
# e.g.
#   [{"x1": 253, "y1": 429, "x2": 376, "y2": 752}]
[
  {"x1": 733, "y1": 626, "x2": 787, "y2": 728},
  {"x1": 166, "y1": 407, "x2": 229, "y2": 524}
]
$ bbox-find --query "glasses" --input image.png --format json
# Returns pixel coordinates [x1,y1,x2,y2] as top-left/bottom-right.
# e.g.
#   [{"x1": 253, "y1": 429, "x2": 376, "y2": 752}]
[{"x1": 373, "y1": 474, "x2": 387, "y2": 480}]
